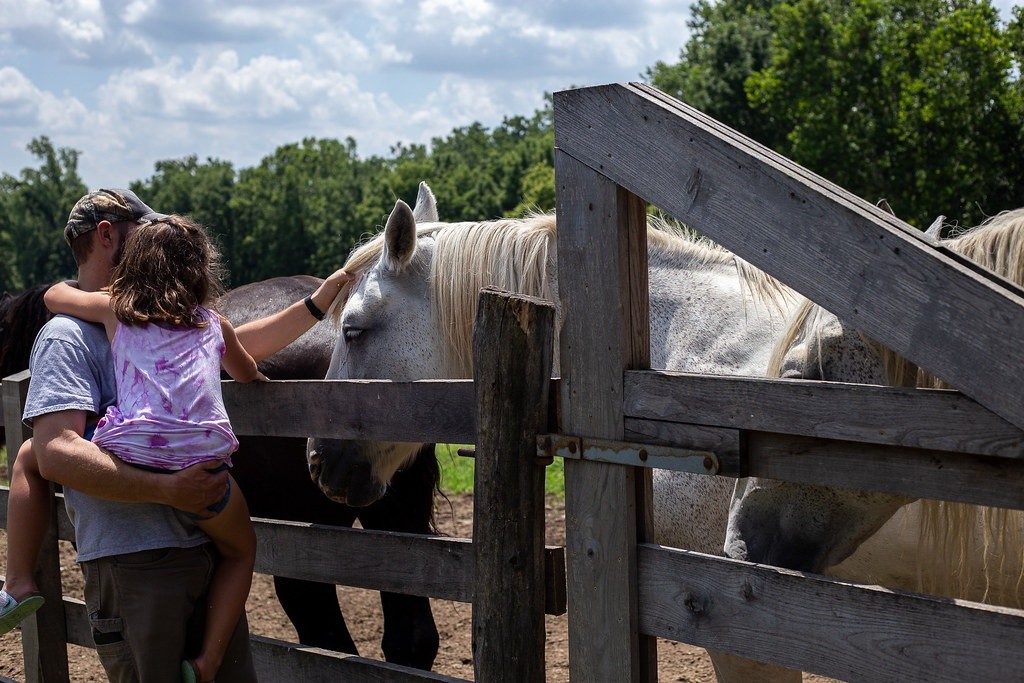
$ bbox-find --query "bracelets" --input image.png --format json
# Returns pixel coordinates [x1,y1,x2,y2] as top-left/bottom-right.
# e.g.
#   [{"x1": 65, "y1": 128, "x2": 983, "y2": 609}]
[{"x1": 303, "y1": 295, "x2": 326, "y2": 321}]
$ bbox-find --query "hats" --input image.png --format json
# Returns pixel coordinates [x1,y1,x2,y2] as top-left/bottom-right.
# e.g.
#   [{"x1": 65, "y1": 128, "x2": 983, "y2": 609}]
[{"x1": 67, "y1": 189, "x2": 169, "y2": 239}]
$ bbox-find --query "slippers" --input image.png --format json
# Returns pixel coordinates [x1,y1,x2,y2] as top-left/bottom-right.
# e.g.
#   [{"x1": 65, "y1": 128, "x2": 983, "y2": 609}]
[
  {"x1": 182, "y1": 659, "x2": 215, "y2": 683},
  {"x1": 0, "y1": 589, "x2": 46, "y2": 636}
]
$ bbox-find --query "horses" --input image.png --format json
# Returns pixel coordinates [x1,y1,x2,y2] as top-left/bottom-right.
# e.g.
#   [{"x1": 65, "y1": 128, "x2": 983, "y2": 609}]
[
  {"x1": 0, "y1": 273, "x2": 456, "y2": 672},
  {"x1": 307, "y1": 183, "x2": 1024, "y2": 682}
]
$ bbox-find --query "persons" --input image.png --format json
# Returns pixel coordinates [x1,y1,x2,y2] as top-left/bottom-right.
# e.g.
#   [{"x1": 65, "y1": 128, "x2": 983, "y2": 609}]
[
  {"x1": 22, "y1": 185, "x2": 354, "y2": 683},
  {"x1": 0, "y1": 214, "x2": 270, "y2": 683}
]
